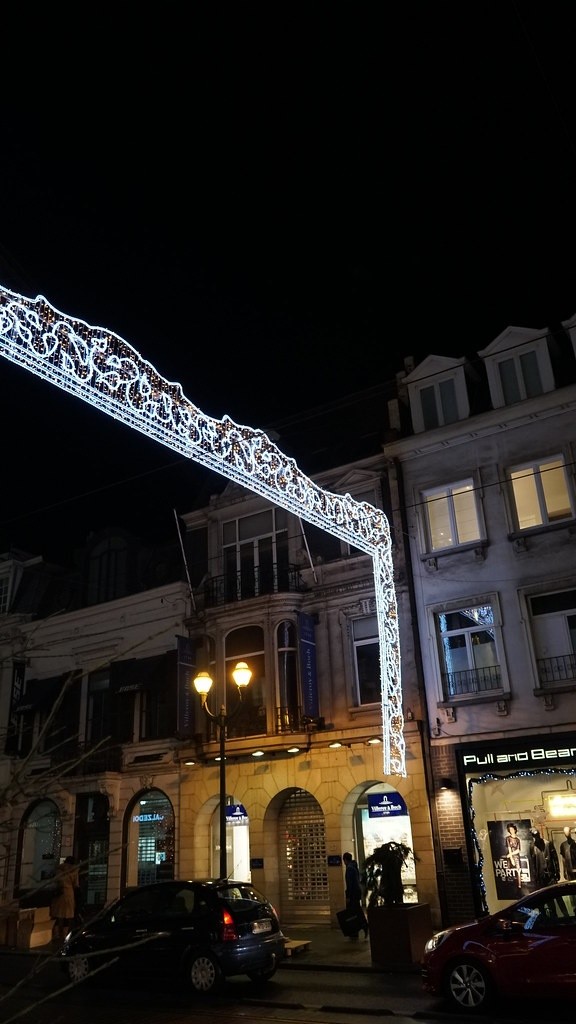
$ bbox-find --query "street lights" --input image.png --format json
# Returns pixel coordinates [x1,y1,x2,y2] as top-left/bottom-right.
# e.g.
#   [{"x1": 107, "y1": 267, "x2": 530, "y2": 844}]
[{"x1": 192, "y1": 660, "x2": 252, "y2": 879}]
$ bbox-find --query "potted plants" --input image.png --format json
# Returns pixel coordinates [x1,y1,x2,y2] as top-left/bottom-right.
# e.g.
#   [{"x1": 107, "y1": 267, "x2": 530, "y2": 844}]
[{"x1": 360, "y1": 844, "x2": 434, "y2": 968}]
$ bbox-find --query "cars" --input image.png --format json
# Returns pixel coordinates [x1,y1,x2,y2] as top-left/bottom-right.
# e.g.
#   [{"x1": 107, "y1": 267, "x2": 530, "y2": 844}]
[
  {"x1": 419, "y1": 879, "x2": 575, "y2": 1013},
  {"x1": 58, "y1": 878, "x2": 289, "y2": 995}
]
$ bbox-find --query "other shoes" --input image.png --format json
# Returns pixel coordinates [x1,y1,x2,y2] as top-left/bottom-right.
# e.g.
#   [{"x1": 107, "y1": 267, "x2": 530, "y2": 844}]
[{"x1": 363, "y1": 923, "x2": 369, "y2": 938}]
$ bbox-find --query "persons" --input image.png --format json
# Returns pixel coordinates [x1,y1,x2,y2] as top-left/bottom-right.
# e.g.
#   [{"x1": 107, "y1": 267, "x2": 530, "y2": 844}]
[
  {"x1": 342, "y1": 852, "x2": 369, "y2": 943},
  {"x1": 377, "y1": 842, "x2": 409, "y2": 905},
  {"x1": 529, "y1": 827, "x2": 576, "y2": 917},
  {"x1": 506, "y1": 823, "x2": 521, "y2": 888},
  {"x1": 50, "y1": 856, "x2": 80, "y2": 942},
  {"x1": 471, "y1": 632, "x2": 481, "y2": 645}
]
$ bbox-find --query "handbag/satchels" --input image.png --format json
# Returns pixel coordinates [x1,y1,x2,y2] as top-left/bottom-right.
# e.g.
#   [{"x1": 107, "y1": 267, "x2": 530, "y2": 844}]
[{"x1": 336, "y1": 906, "x2": 367, "y2": 937}]
